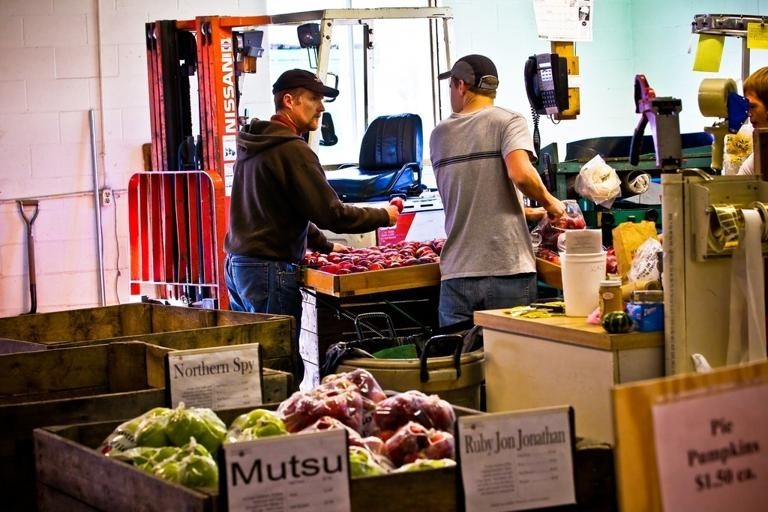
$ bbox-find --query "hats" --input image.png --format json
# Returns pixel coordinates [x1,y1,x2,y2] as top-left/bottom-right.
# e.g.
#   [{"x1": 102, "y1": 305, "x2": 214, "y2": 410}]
[
  {"x1": 438, "y1": 54, "x2": 499, "y2": 91},
  {"x1": 272, "y1": 67, "x2": 340, "y2": 98}
]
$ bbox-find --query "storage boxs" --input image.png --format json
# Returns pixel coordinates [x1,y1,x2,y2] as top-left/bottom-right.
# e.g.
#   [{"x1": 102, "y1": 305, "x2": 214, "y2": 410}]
[{"x1": 0, "y1": 256, "x2": 562, "y2": 512}]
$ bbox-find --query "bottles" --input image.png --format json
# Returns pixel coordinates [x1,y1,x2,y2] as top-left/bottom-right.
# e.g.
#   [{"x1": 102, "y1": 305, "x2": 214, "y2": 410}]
[{"x1": 596, "y1": 279, "x2": 624, "y2": 322}]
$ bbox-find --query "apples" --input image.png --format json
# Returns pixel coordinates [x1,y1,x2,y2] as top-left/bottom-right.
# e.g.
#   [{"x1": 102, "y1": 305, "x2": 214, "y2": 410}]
[
  {"x1": 536, "y1": 247, "x2": 614, "y2": 282},
  {"x1": 390, "y1": 197, "x2": 404, "y2": 213},
  {"x1": 305, "y1": 238, "x2": 446, "y2": 274}
]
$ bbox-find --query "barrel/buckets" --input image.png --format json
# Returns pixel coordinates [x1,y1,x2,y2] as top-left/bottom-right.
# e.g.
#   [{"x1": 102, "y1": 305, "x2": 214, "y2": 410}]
[{"x1": 558, "y1": 245, "x2": 608, "y2": 316}]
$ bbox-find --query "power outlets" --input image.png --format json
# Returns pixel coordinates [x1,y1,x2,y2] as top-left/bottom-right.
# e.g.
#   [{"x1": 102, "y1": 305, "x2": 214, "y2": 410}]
[{"x1": 102, "y1": 188, "x2": 112, "y2": 205}]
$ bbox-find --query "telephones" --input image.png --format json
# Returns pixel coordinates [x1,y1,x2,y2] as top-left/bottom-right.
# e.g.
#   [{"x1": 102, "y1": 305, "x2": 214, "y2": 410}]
[{"x1": 524, "y1": 53, "x2": 569, "y2": 114}]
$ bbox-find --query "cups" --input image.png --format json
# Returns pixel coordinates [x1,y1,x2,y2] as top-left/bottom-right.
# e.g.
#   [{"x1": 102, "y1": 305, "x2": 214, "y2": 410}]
[{"x1": 559, "y1": 248, "x2": 608, "y2": 319}]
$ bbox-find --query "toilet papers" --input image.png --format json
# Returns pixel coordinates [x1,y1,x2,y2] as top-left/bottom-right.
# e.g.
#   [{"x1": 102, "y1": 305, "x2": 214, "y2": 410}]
[{"x1": 698, "y1": 76, "x2": 738, "y2": 118}]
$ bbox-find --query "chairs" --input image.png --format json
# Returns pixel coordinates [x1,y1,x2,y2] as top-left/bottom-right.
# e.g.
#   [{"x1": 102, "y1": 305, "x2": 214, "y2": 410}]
[{"x1": 325, "y1": 113, "x2": 422, "y2": 202}]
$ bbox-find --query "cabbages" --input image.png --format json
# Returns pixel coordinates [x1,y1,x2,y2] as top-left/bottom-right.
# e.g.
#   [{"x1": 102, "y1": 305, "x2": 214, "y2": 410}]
[{"x1": 98, "y1": 404, "x2": 456, "y2": 489}]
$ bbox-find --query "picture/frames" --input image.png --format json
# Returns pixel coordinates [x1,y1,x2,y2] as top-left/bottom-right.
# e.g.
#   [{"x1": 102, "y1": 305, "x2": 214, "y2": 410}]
[{"x1": 611, "y1": 361, "x2": 767, "y2": 511}]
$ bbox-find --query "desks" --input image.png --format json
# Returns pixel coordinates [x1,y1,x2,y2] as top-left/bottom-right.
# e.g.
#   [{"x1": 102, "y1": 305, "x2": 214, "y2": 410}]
[
  {"x1": 534, "y1": 155, "x2": 713, "y2": 202},
  {"x1": 473, "y1": 307, "x2": 665, "y2": 446}
]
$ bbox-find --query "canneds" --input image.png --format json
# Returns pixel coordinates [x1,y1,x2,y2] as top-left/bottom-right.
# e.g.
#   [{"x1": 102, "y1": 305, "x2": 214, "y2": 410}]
[{"x1": 599, "y1": 282, "x2": 623, "y2": 325}]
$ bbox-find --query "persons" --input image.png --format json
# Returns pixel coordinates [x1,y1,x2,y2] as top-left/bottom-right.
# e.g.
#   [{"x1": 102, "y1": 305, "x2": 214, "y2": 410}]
[
  {"x1": 736, "y1": 66, "x2": 768, "y2": 176},
  {"x1": 429, "y1": 54, "x2": 569, "y2": 334},
  {"x1": 224, "y1": 69, "x2": 400, "y2": 400}
]
